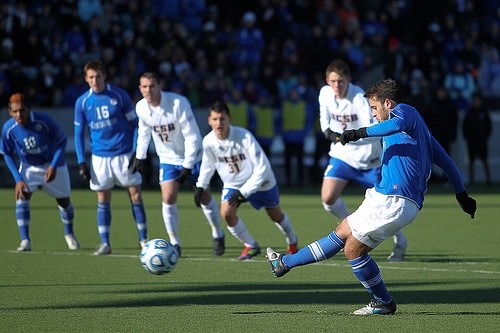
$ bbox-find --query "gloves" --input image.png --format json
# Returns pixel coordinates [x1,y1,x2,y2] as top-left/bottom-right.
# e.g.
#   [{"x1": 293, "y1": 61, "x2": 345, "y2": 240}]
[
  {"x1": 79, "y1": 162, "x2": 90, "y2": 183},
  {"x1": 455, "y1": 190, "x2": 477, "y2": 219},
  {"x1": 175, "y1": 167, "x2": 192, "y2": 183},
  {"x1": 329, "y1": 131, "x2": 342, "y2": 144},
  {"x1": 127, "y1": 152, "x2": 136, "y2": 169},
  {"x1": 228, "y1": 190, "x2": 246, "y2": 209},
  {"x1": 131, "y1": 159, "x2": 145, "y2": 175},
  {"x1": 340, "y1": 126, "x2": 369, "y2": 146},
  {"x1": 194, "y1": 187, "x2": 204, "y2": 208}
]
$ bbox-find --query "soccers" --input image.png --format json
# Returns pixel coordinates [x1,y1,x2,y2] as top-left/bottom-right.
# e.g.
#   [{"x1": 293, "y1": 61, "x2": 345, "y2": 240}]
[{"x1": 140, "y1": 239, "x2": 178, "y2": 275}]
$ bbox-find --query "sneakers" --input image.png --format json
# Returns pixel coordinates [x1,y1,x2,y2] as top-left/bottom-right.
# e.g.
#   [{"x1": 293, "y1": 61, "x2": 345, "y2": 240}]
[
  {"x1": 17, "y1": 239, "x2": 31, "y2": 251},
  {"x1": 353, "y1": 296, "x2": 397, "y2": 315},
  {"x1": 387, "y1": 238, "x2": 406, "y2": 261},
  {"x1": 140, "y1": 240, "x2": 146, "y2": 248},
  {"x1": 65, "y1": 234, "x2": 80, "y2": 250},
  {"x1": 265, "y1": 247, "x2": 290, "y2": 278},
  {"x1": 94, "y1": 244, "x2": 111, "y2": 255}
]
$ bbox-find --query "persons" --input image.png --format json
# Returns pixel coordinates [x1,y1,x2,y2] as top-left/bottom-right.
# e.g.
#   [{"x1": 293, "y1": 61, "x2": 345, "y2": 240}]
[
  {"x1": 0, "y1": 92, "x2": 85, "y2": 252},
  {"x1": 265, "y1": 80, "x2": 476, "y2": 315},
  {"x1": 192, "y1": 101, "x2": 298, "y2": 260},
  {"x1": 73, "y1": 59, "x2": 150, "y2": 256},
  {"x1": 128, "y1": 72, "x2": 224, "y2": 256},
  {"x1": 318, "y1": 60, "x2": 407, "y2": 262},
  {"x1": 0, "y1": 0, "x2": 500, "y2": 188}
]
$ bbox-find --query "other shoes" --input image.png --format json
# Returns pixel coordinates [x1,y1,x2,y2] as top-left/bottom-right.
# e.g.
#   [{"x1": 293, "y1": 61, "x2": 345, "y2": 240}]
[
  {"x1": 173, "y1": 244, "x2": 182, "y2": 257},
  {"x1": 287, "y1": 237, "x2": 298, "y2": 255},
  {"x1": 238, "y1": 242, "x2": 261, "y2": 260},
  {"x1": 212, "y1": 233, "x2": 225, "y2": 255}
]
